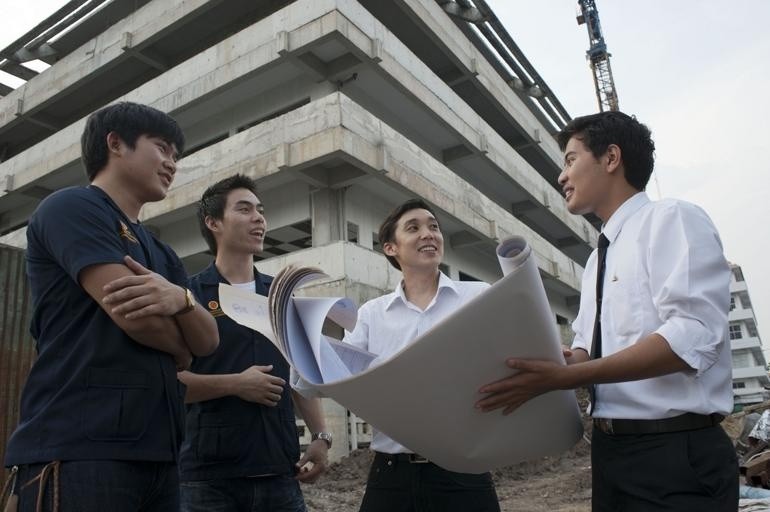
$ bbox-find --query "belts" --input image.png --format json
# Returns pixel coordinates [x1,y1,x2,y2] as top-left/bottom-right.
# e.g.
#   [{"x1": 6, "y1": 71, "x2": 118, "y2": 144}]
[
  {"x1": 595, "y1": 413, "x2": 726, "y2": 435},
  {"x1": 376, "y1": 451, "x2": 431, "y2": 465}
]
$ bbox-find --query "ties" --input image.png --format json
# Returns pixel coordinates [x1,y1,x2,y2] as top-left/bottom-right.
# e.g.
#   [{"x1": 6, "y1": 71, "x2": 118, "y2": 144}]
[{"x1": 587, "y1": 232, "x2": 610, "y2": 416}]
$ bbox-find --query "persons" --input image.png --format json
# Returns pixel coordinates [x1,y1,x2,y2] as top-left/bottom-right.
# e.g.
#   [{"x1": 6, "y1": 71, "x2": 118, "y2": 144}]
[
  {"x1": 170, "y1": 171, "x2": 332, "y2": 511},
  {"x1": 471, "y1": 109, "x2": 741, "y2": 512},
  {"x1": 0, "y1": 100, "x2": 221, "y2": 512},
  {"x1": 336, "y1": 197, "x2": 500, "y2": 512}
]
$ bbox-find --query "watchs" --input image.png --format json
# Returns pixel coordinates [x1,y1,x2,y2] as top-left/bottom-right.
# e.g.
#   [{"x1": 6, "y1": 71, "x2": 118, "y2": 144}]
[
  {"x1": 176, "y1": 286, "x2": 195, "y2": 317},
  {"x1": 311, "y1": 432, "x2": 333, "y2": 449}
]
